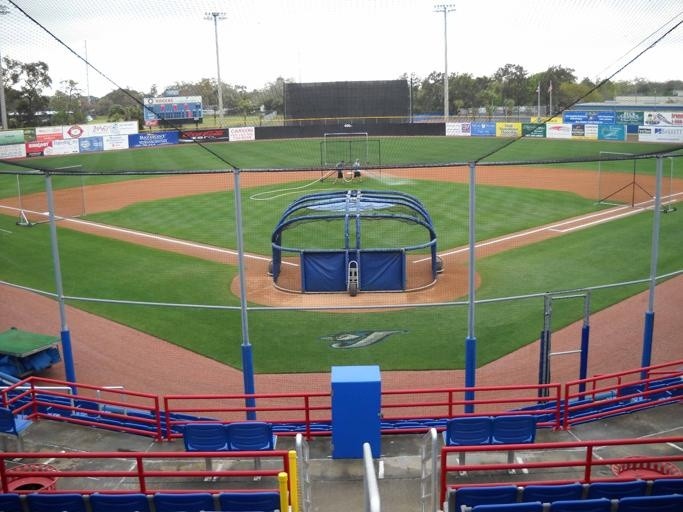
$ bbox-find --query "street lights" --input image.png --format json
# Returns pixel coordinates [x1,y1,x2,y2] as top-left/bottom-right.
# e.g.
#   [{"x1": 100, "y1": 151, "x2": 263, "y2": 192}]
[
  {"x1": 203, "y1": 10, "x2": 227, "y2": 126},
  {"x1": 433, "y1": 2, "x2": 457, "y2": 119}
]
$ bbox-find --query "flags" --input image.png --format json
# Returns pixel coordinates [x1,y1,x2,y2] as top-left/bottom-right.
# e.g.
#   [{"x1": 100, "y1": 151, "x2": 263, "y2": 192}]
[
  {"x1": 535, "y1": 85, "x2": 539, "y2": 93},
  {"x1": 548, "y1": 82, "x2": 553, "y2": 93}
]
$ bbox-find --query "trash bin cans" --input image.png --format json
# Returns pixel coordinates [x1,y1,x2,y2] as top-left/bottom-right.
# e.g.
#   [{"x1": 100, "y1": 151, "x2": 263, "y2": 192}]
[
  {"x1": 611, "y1": 455, "x2": 682, "y2": 477},
  {"x1": 0, "y1": 462, "x2": 57, "y2": 495}
]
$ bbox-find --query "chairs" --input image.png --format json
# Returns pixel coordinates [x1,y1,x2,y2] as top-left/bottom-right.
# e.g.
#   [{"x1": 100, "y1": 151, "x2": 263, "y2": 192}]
[
  {"x1": 441, "y1": 415, "x2": 538, "y2": 476},
  {"x1": 0, "y1": 408, "x2": 34, "y2": 461},
  {"x1": 449, "y1": 478, "x2": 683, "y2": 511},
  {"x1": 184, "y1": 420, "x2": 278, "y2": 484},
  {"x1": 0, "y1": 370, "x2": 681, "y2": 437},
  {"x1": 0, "y1": 491, "x2": 281, "y2": 511}
]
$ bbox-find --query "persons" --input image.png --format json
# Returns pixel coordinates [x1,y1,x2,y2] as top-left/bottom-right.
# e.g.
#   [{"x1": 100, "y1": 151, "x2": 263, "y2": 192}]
[
  {"x1": 332, "y1": 160, "x2": 346, "y2": 185},
  {"x1": 352, "y1": 158, "x2": 363, "y2": 184}
]
